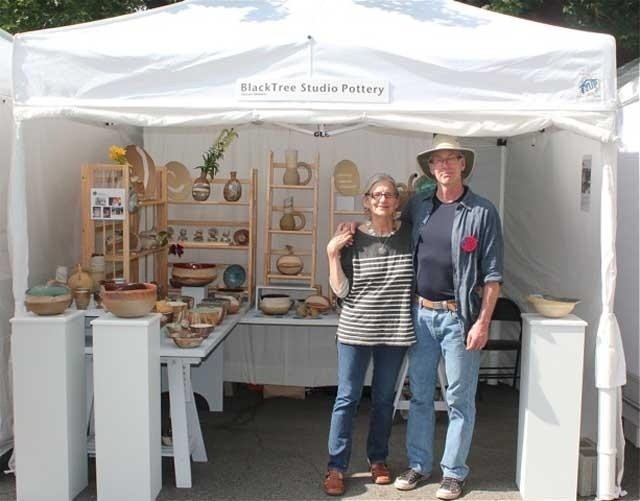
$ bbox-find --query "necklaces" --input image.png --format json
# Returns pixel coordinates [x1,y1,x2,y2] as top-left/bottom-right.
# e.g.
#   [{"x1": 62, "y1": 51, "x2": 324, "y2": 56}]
[{"x1": 367, "y1": 223, "x2": 397, "y2": 254}]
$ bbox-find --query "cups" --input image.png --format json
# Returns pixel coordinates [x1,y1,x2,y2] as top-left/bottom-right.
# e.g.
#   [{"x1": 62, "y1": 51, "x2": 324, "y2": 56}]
[{"x1": 74, "y1": 287, "x2": 91, "y2": 310}]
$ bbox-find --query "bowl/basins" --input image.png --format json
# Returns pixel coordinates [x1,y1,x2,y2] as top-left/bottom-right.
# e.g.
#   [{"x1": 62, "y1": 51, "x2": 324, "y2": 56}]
[
  {"x1": 100, "y1": 283, "x2": 159, "y2": 318},
  {"x1": 26, "y1": 284, "x2": 73, "y2": 317},
  {"x1": 191, "y1": 323, "x2": 213, "y2": 337},
  {"x1": 526, "y1": 296, "x2": 581, "y2": 319},
  {"x1": 161, "y1": 294, "x2": 231, "y2": 322},
  {"x1": 260, "y1": 292, "x2": 291, "y2": 316},
  {"x1": 172, "y1": 263, "x2": 217, "y2": 287}
]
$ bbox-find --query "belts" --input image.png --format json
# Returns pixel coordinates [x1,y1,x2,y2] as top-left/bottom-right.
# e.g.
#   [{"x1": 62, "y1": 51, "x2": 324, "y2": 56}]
[{"x1": 413, "y1": 295, "x2": 456, "y2": 309}]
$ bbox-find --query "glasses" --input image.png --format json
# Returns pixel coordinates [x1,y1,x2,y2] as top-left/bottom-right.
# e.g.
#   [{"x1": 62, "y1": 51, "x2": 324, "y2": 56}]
[{"x1": 370, "y1": 192, "x2": 395, "y2": 198}]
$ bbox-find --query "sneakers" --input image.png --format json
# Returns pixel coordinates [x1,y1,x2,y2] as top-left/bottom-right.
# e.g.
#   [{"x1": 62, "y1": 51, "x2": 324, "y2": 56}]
[
  {"x1": 394, "y1": 470, "x2": 431, "y2": 490},
  {"x1": 366, "y1": 458, "x2": 391, "y2": 484},
  {"x1": 435, "y1": 477, "x2": 465, "y2": 499},
  {"x1": 323, "y1": 469, "x2": 345, "y2": 495}
]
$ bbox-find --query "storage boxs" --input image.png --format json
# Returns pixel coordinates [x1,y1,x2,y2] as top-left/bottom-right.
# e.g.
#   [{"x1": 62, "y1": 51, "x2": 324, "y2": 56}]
[{"x1": 263, "y1": 383, "x2": 304, "y2": 400}]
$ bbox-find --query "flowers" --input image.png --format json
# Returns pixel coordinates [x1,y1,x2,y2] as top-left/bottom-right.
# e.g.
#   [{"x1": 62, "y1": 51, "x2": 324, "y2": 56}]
[
  {"x1": 191, "y1": 129, "x2": 240, "y2": 180},
  {"x1": 109, "y1": 144, "x2": 128, "y2": 164}
]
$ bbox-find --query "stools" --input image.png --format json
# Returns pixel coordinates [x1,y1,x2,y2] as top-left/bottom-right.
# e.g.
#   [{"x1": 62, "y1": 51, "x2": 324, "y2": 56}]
[{"x1": 392, "y1": 352, "x2": 450, "y2": 420}]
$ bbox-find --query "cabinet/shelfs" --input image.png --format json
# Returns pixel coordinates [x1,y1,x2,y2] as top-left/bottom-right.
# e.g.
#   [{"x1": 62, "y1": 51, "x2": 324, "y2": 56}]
[
  {"x1": 167, "y1": 168, "x2": 257, "y2": 304},
  {"x1": 266, "y1": 151, "x2": 322, "y2": 287},
  {"x1": 80, "y1": 164, "x2": 169, "y2": 299},
  {"x1": 330, "y1": 177, "x2": 404, "y2": 305}
]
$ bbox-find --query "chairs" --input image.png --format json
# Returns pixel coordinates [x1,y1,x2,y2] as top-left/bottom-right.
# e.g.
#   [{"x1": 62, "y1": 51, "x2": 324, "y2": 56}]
[{"x1": 476, "y1": 298, "x2": 523, "y2": 396}]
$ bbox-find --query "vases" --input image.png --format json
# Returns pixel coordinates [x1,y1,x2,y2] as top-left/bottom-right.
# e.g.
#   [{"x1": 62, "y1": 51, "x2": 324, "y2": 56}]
[
  {"x1": 276, "y1": 245, "x2": 304, "y2": 276},
  {"x1": 129, "y1": 184, "x2": 137, "y2": 213},
  {"x1": 193, "y1": 168, "x2": 211, "y2": 201},
  {"x1": 223, "y1": 171, "x2": 242, "y2": 201}
]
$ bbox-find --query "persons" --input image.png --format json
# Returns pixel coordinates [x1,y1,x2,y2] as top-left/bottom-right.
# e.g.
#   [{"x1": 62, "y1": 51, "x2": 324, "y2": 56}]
[
  {"x1": 325, "y1": 171, "x2": 420, "y2": 495},
  {"x1": 395, "y1": 132, "x2": 503, "y2": 501}
]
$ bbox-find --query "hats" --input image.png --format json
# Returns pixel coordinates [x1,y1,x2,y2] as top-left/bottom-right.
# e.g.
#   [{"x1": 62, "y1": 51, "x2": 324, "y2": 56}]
[{"x1": 416, "y1": 134, "x2": 475, "y2": 182}]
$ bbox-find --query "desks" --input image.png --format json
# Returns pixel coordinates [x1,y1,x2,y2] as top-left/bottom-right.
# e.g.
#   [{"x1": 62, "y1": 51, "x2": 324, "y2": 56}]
[
  {"x1": 70, "y1": 293, "x2": 253, "y2": 489},
  {"x1": 222, "y1": 299, "x2": 374, "y2": 399}
]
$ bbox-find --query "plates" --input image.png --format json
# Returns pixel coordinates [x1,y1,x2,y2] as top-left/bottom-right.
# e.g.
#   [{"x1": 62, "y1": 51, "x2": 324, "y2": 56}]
[
  {"x1": 224, "y1": 264, "x2": 246, "y2": 290},
  {"x1": 165, "y1": 162, "x2": 192, "y2": 200},
  {"x1": 125, "y1": 144, "x2": 156, "y2": 200},
  {"x1": 234, "y1": 230, "x2": 249, "y2": 247},
  {"x1": 166, "y1": 334, "x2": 205, "y2": 349},
  {"x1": 334, "y1": 160, "x2": 361, "y2": 197},
  {"x1": 103, "y1": 232, "x2": 144, "y2": 255}
]
